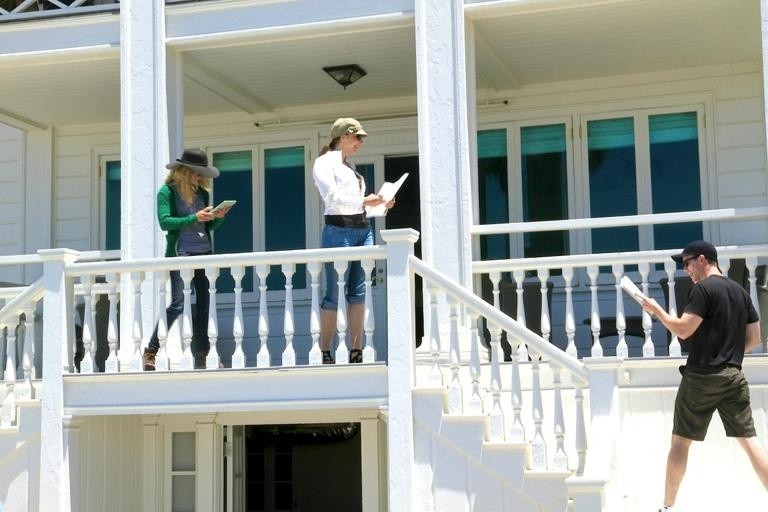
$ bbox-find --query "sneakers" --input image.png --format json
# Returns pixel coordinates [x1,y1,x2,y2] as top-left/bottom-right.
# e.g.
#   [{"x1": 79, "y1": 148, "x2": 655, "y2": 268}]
[
  {"x1": 348, "y1": 349, "x2": 364, "y2": 363},
  {"x1": 319, "y1": 349, "x2": 336, "y2": 363},
  {"x1": 143, "y1": 350, "x2": 155, "y2": 371},
  {"x1": 193, "y1": 351, "x2": 225, "y2": 370}
]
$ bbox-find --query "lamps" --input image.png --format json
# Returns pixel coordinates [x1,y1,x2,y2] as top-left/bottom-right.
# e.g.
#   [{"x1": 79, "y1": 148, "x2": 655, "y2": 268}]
[
  {"x1": 322, "y1": 64, "x2": 367, "y2": 90},
  {"x1": 140, "y1": 415, "x2": 168, "y2": 426}
]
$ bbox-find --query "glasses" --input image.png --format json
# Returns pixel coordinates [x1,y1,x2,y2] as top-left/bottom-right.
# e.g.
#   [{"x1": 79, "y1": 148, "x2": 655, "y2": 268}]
[
  {"x1": 682, "y1": 256, "x2": 699, "y2": 268},
  {"x1": 353, "y1": 135, "x2": 361, "y2": 141}
]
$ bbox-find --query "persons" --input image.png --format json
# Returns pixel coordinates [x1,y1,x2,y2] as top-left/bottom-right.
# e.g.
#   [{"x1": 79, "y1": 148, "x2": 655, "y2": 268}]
[
  {"x1": 630, "y1": 238, "x2": 767, "y2": 512},
  {"x1": 132, "y1": 143, "x2": 240, "y2": 372},
  {"x1": 310, "y1": 116, "x2": 397, "y2": 363}
]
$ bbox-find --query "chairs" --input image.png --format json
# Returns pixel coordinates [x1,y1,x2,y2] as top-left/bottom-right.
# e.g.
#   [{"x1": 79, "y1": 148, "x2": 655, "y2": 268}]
[
  {"x1": 659, "y1": 257, "x2": 750, "y2": 354},
  {"x1": 756, "y1": 265, "x2": 768, "y2": 353},
  {"x1": 482, "y1": 257, "x2": 553, "y2": 361}
]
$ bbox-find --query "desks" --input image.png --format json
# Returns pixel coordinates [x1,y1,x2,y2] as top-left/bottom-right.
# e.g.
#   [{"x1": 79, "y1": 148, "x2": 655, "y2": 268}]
[{"x1": 584, "y1": 317, "x2": 657, "y2": 344}]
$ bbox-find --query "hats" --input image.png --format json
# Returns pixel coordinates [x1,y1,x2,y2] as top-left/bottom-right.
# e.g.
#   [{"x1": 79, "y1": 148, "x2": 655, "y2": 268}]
[
  {"x1": 331, "y1": 117, "x2": 367, "y2": 139},
  {"x1": 163, "y1": 148, "x2": 220, "y2": 179},
  {"x1": 670, "y1": 240, "x2": 718, "y2": 264}
]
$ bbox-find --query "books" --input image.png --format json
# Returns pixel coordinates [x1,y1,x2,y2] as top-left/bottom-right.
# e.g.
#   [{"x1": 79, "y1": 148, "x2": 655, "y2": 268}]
[
  {"x1": 210, "y1": 199, "x2": 237, "y2": 216},
  {"x1": 620, "y1": 274, "x2": 656, "y2": 316}
]
